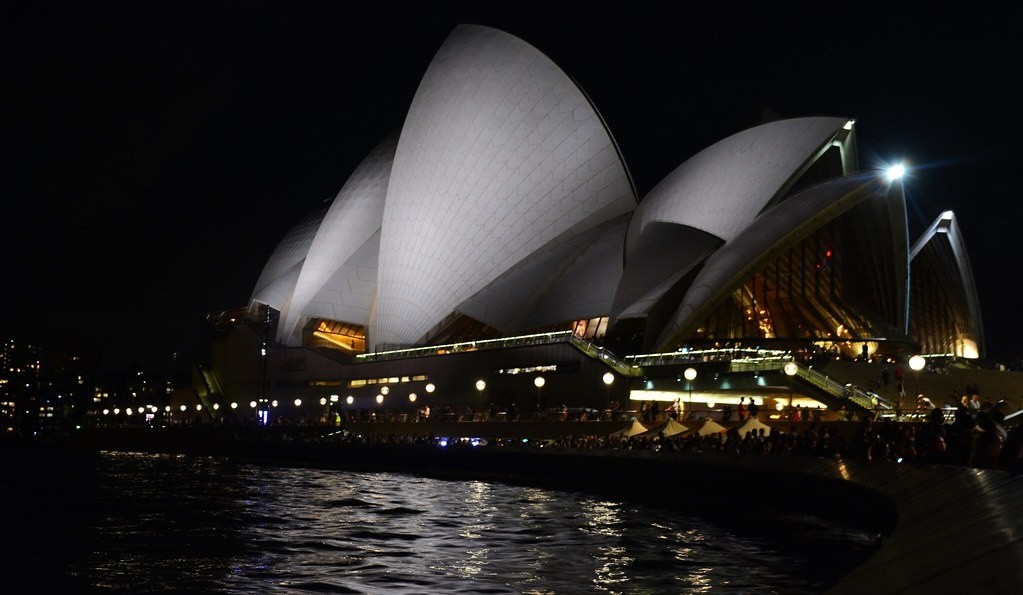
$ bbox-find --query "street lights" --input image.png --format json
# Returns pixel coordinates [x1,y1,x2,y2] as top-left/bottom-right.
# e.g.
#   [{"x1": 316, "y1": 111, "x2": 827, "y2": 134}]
[
  {"x1": 909, "y1": 355, "x2": 926, "y2": 420},
  {"x1": 683, "y1": 368, "x2": 698, "y2": 418},
  {"x1": 381, "y1": 386, "x2": 390, "y2": 424},
  {"x1": 602, "y1": 372, "x2": 615, "y2": 416},
  {"x1": 424, "y1": 382, "x2": 437, "y2": 420},
  {"x1": 475, "y1": 380, "x2": 487, "y2": 414},
  {"x1": 534, "y1": 377, "x2": 546, "y2": 414},
  {"x1": 784, "y1": 362, "x2": 798, "y2": 413}
]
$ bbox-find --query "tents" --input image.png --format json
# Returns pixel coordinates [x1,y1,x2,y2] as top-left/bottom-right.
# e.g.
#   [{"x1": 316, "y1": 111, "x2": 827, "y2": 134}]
[
  {"x1": 662, "y1": 417, "x2": 690, "y2": 437},
  {"x1": 612, "y1": 417, "x2": 648, "y2": 439},
  {"x1": 738, "y1": 419, "x2": 772, "y2": 440},
  {"x1": 698, "y1": 416, "x2": 728, "y2": 437}
]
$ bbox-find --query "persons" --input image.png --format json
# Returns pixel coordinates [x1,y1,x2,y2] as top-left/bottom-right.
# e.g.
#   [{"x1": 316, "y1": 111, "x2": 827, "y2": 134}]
[
  {"x1": 898, "y1": 379, "x2": 907, "y2": 399},
  {"x1": 269, "y1": 384, "x2": 1014, "y2": 462},
  {"x1": 896, "y1": 367, "x2": 902, "y2": 379},
  {"x1": 714, "y1": 342, "x2": 760, "y2": 361},
  {"x1": 881, "y1": 367, "x2": 889, "y2": 387},
  {"x1": 863, "y1": 342, "x2": 868, "y2": 358},
  {"x1": 670, "y1": 344, "x2": 690, "y2": 359}
]
